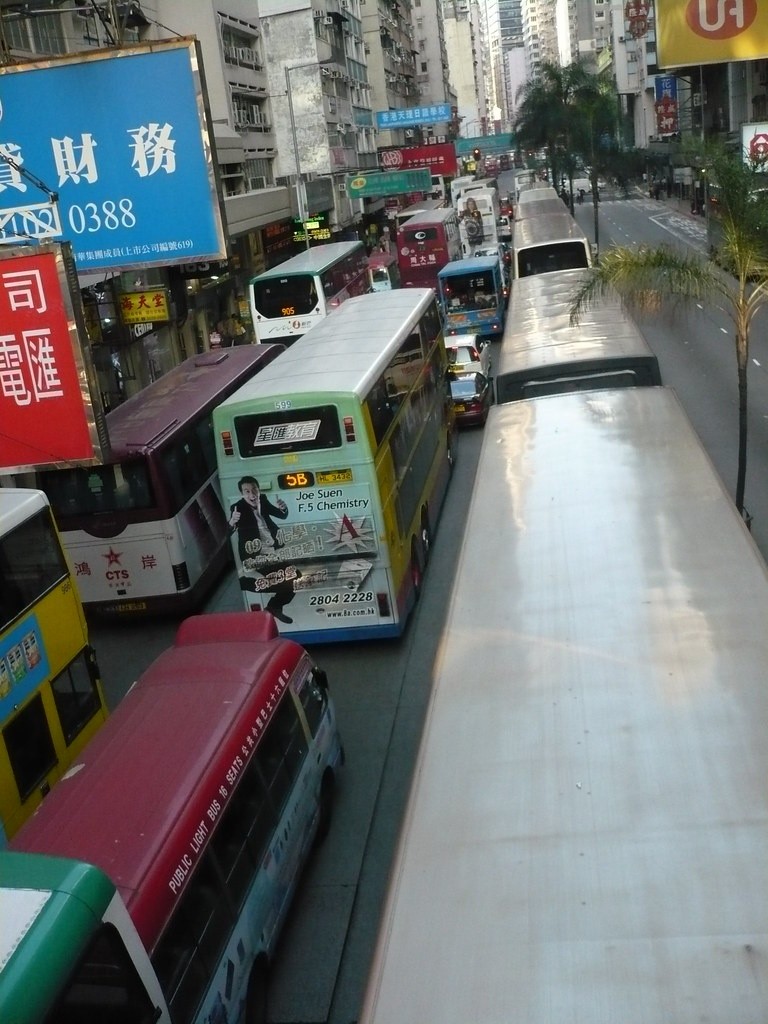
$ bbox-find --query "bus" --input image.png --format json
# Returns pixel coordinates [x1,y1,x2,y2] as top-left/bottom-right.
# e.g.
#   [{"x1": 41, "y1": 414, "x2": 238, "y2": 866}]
[
  {"x1": 0, "y1": 848, "x2": 173, "y2": 1024},
  {"x1": 434, "y1": 255, "x2": 508, "y2": 340},
  {"x1": 0, "y1": 611, "x2": 349, "y2": 1024},
  {"x1": 491, "y1": 267, "x2": 664, "y2": 402},
  {"x1": 369, "y1": 145, "x2": 614, "y2": 300},
  {"x1": 33, "y1": 343, "x2": 290, "y2": 617},
  {"x1": 362, "y1": 385, "x2": 768, "y2": 1023},
  {"x1": 0, "y1": 483, "x2": 111, "y2": 854},
  {"x1": 211, "y1": 285, "x2": 456, "y2": 642},
  {"x1": 248, "y1": 239, "x2": 373, "y2": 346}
]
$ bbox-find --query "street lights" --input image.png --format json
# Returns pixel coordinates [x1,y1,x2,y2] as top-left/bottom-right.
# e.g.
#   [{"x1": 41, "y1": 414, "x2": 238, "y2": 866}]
[{"x1": 283, "y1": 56, "x2": 340, "y2": 247}]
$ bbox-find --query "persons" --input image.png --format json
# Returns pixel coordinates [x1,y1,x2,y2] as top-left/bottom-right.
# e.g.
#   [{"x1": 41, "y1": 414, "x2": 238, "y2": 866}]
[
  {"x1": 690, "y1": 200, "x2": 706, "y2": 217},
  {"x1": 613, "y1": 176, "x2": 618, "y2": 188},
  {"x1": 539, "y1": 167, "x2": 547, "y2": 182},
  {"x1": 643, "y1": 173, "x2": 668, "y2": 199},
  {"x1": 364, "y1": 234, "x2": 387, "y2": 253},
  {"x1": 226, "y1": 475, "x2": 303, "y2": 623},
  {"x1": 454, "y1": 197, "x2": 485, "y2": 257},
  {"x1": 215, "y1": 314, "x2": 245, "y2": 345},
  {"x1": 560, "y1": 185, "x2": 584, "y2": 206}
]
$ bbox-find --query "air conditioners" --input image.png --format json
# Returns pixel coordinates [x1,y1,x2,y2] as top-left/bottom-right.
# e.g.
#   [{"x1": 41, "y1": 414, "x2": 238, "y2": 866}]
[
  {"x1": 406, "y1": 130, "x2": 415, "y2": 139},
  {"x1": 340, "y1": 183, "x2": 347, "y2": 192},
  {"x1": 396, "y1": 42, "x2": 404, "y2": 48},
  {"x1": 337, "y1": 123, "x2": 378, "y2": 135},
  {"x1": 228, "y1": 46, "x2": 259, "y2": 63},
  {"x1": 232, "y1": 101, "x2": 266, "y2": 123},
  {"x1": 316, "y1": 9, "x2": 333, "y2": 25},
  {"x1": 323, "y1": 68, "x2": 371, "y2": 90},
  {"x1": 390, "y1": 77, "x2": 396, "y2": 83},
  {"x1": 391, "y1": 4, "x2": 396, "y2": 9}
]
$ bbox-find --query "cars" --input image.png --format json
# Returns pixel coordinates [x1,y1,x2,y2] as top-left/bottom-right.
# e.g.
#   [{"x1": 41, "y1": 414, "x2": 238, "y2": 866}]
[
  {"x1": 448, "y1": 370, "x2": 494, "y2": 428},
  {"x1": 443, "y1": 334, "x2": 492, "y2": 382}
]
